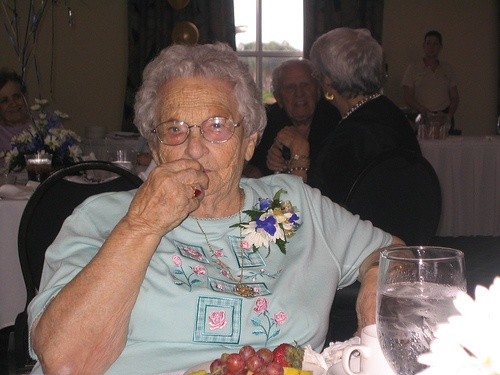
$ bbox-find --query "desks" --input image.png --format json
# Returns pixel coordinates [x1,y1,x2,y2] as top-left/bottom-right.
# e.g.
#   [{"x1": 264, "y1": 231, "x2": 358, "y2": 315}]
[
  {"x1": 419, "y1": 134, "x2": 500, "y2": 238},
  {"x1": 0, "y1": 164, "x2": 149, "y2": 329}
]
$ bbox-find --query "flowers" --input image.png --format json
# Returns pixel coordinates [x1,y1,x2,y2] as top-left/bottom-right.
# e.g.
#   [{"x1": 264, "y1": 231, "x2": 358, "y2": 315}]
[
  {"x1": 229, "y1": 187, "x2": 305, "y2": 255},
  {"x1": 0, "y1": 98, "x2": 84, "y2": 175}
]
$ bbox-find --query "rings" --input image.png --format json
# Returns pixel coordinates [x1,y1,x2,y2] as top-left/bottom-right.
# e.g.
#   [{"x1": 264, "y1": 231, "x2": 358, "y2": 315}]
[{"x1": 190, "y1": 185, "x2": 202, "y2": 198}]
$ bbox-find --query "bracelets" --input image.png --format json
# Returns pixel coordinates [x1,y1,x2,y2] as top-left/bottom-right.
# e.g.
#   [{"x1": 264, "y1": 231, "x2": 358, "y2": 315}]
[
  {"x1": 288, "y1": 167, "x2": 309, "y2": 173},
  {"x1": 290, "y1": 154, "x2": 310, "y2": 160}
]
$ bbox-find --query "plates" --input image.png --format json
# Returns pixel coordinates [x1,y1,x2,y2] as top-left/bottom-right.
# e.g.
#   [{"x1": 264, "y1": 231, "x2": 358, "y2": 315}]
[{"x1": 326, "y1": 358, "x2": 362, "y2": 375}]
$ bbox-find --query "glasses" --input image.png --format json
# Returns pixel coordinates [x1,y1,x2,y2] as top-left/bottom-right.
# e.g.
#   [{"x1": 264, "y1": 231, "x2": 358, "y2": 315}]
[{"x1": 150, "y1": 116, "x2": 244, "y2": 146}]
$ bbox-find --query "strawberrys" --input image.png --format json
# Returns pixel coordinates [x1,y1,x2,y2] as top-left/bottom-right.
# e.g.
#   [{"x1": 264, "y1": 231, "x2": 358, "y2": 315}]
[{"x1": 273, "y1": 343, "x2": 293, "y2": 367}]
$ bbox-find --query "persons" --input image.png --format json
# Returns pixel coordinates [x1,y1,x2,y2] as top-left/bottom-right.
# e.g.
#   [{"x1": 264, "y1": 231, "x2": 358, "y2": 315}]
[
  {"x1": 27, "y1": 40, "x2": 415, "y2": 375},
  {"x1": 0, "y1": 67, "x2": 35, "y2": 156},
  {"x1": 401, "y1": 31, "x2": 460, "y2": 130},
  {"x1": 275, "y1": 27, "x2": 441, "y2": 354},
  {"x1": 243, "y1": 58, "x2": 338, "y2": 180}
]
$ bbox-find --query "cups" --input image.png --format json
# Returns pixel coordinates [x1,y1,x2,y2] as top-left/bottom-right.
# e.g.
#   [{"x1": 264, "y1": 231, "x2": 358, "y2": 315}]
[
  {"x1": 78, "y1": 124, "x2": 145, "y2": 178},
  {"x1": 417, "y1": 111, "x2": 450, "y2": 142},
  {"x1": 24, "y1": 154, "x2": 53, "y2": 183},
  {"x1": 342, "y1": 324, "x2": 397, "y2": 375},
  {"x1": 0, "y1": 163, "x2": 18, "y2": 186},
  {"x1": 376, "y1": 246, "x2": 467, "y2": 375}
]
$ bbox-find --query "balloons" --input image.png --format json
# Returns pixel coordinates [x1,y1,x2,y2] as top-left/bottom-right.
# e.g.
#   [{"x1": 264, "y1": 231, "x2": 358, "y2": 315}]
[
  {"x1": 167, "y1": 0, "x2": 189, "y2": 10},
  {"x1": 171, "y1": 21, "x2": 199, "y2": 45}
]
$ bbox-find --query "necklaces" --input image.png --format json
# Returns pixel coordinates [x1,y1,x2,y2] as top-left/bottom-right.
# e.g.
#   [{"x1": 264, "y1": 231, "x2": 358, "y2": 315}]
[
  {"x1": 194, "y1": 188, "x2": 254, "y2": 296},
  {"x1": 339, "y1": 93, "x2": 381, "y2": 122}
]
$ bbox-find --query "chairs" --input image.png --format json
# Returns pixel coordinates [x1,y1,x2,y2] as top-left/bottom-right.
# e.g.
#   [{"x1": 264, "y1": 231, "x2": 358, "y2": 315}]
[
  {"x1": 342, "y1": 149, "x2": 441, "y2": 246},
  {"x1": 5, "y1": 160, "x2": 144, "y2": 375}
]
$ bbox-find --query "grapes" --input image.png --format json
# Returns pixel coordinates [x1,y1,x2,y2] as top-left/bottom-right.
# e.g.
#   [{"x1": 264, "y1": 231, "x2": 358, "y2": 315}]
[{"x1": 210, "y1": 345, "x2": 284, "y2": 375}]
